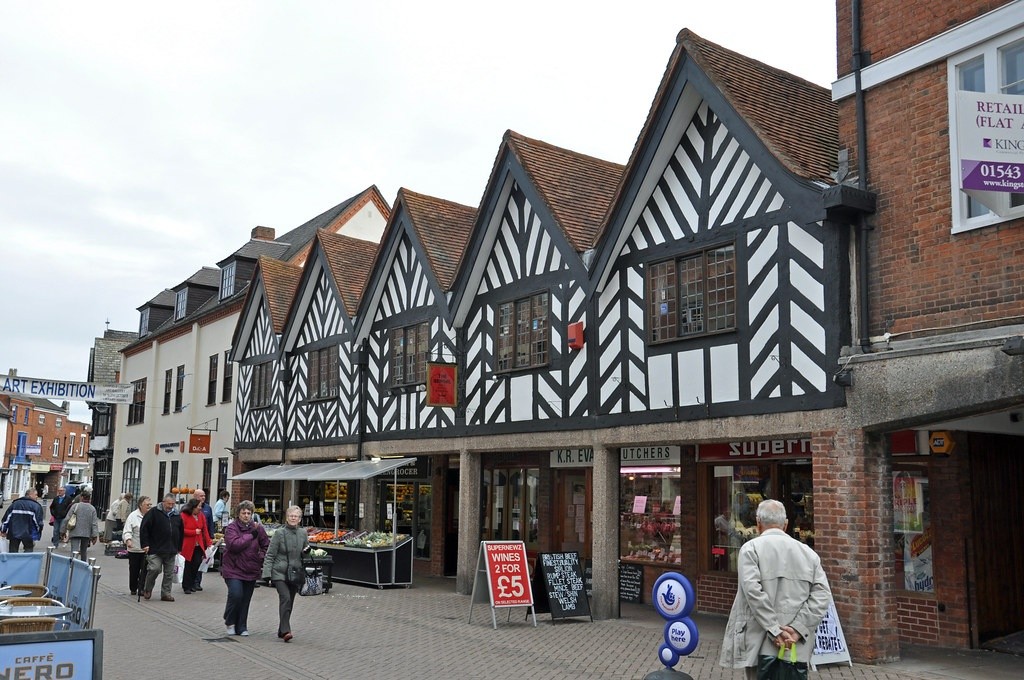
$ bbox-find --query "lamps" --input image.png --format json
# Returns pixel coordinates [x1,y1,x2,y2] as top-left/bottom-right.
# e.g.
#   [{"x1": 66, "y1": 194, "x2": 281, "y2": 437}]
[
  {"x1": 224, "y1": 447, "x2": 242, "y2": 455},
  {"x1": 92, "y1": 402, "x2": 111, "y2": 413}
]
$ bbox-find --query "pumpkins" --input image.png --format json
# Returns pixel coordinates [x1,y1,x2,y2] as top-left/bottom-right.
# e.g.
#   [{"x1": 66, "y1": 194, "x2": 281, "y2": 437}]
[{"x1": 172, "y1": 487, "x2": 196, "y2": 494}]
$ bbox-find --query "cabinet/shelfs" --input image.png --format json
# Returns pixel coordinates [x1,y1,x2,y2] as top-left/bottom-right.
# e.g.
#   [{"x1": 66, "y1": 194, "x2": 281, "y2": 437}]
[
  {"x1": 325, "y1": 482, "x2": 432, "y2": 515},
  {"x1": 255, "y1": 503, "x2": 281, "y2": 515}
]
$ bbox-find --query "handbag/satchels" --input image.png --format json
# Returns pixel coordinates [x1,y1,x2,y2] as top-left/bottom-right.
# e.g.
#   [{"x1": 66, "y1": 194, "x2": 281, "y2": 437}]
[
  {"x1": 298, "y1": 552, "x2": 323, "y2": 596},
  {"x1": 205, "y1": 544, "x2": 217, "y2": 568},
  {"x1": 287, "y1": 565, "x2": 306, "y2": 587},
  {"x1": 67, "y1": 503, "x2": 79, "y2": 530},
  {"x1": 757, "y1": 641, "x2": 808, "y2": 680},
  {"x1": 173, "y1": 553, "x2": 185, "y2": 584}
]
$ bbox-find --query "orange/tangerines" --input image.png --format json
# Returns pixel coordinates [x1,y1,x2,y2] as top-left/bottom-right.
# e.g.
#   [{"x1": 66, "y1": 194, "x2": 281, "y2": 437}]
[
  {"x1": 397, "y1": 486, "x2": 428, "y2": 501},
  {"x1": 308, "y1": 530, "x2": 344, "y2": 541}
]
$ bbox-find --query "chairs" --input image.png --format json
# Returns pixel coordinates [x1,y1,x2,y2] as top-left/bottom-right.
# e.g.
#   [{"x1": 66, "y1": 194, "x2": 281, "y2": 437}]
[
  {"x1": 0, "y1": 597, "x2": 71, "y2": 634},
  {"x1": 0, "y1": 585, "x2": 49, "y2": 598}
]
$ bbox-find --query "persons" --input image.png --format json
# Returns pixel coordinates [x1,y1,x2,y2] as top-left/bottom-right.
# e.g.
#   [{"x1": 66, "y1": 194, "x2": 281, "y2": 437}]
[
  {"x1": 41, "y1": 482, "x2": 49, "y2": 499},
  {"x1": 741, "y1": 496, "x2": 757, "y2": 526},
  {"x1": 720, "y1": 498, "x2": 830, "y2": 680},
  {"x1": 36, "y1": 480, "x2": 43, "y2": 497},
  {"x1": 714, "y1": 505, "x2": 736, "y2": 545},
  {"x1": 261, "y1": 503, "x2": 311, "y2": 642},
  {"x1": 121, "y1": 495, "x2": 154, "y2": 595},
  {"x1": 657, "y1": 497, "x2": 676, "y2": 548},
  {"x1": 220, "y1": 500, "x2": 271, "y2": 636},
  {"x1": 180, "y1": 489, "x2": 217, "y2": 592},
  {"x1": 47, "y1": 487, "x2": 72, "y2": 549},
  {"x1": 139, "y1": 492, "x2": 184, "y2": 603},
  {"x1": 58, "y1": 484, "x2": 100, "y2": 561},
  {"x1": 102, "y1": 492, "x2": 134, "y2": 543},
  {"x1": 212, "y1": 491, "x2": 230, "y2": 534},
  {"x1": 179, "y1": 497, "x2": 213, "y2": 594},
  {"x1": 0, "y1": 486, "x2": 43, "y2": 553}
]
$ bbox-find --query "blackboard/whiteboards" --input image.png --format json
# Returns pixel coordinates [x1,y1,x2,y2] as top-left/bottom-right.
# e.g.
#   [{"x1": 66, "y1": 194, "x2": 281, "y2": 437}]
[
  {"x1": 527, "y1": 551, "x2": 594, "y2": 619},
  {"x1": 584, "y1": 558, "x2": 592, "y2": 597}
]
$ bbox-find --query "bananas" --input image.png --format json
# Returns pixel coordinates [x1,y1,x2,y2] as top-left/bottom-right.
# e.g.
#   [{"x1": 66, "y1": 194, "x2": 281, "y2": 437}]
[{"x1": 254, "y1": 507, "x2": 272, "y2": 523}]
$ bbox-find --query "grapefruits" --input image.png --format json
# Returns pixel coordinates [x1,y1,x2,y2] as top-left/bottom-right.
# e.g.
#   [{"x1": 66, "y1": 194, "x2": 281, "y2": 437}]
[{"x1": 324, "y1": 484, "x2": 347, "y2": 499}]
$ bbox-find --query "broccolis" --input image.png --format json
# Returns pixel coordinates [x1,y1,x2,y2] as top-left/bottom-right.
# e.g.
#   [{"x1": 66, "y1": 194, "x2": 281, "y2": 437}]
[{"x1": 309, "y1": 549, "x2": 327, "y2": 557}]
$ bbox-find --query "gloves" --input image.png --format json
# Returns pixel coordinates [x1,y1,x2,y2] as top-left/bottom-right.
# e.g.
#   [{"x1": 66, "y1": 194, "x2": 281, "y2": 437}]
[{"x1": 252, "y1": 530, "x2": 259, "y2": 540}]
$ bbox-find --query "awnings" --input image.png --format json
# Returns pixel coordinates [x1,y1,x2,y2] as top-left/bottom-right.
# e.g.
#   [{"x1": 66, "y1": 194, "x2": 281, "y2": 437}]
[
  {"x1": 265, "y1": 461, "x2": 353, "y2": 538},
  {"x1": 226, "y1": 461, "x2": 310, "y2": 507},
  {"x1": 309, "y1": 458, "x2": 419, "y2": 543}
]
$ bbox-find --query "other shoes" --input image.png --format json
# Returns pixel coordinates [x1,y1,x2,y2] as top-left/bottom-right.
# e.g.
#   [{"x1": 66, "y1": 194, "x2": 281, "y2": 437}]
[
  {"x1": 131, "y1": 591, "x2": 136, "y2": 595},
  {"x1": 225, "y1": 625, "x2": 236, "y2": 635},
  {"x1": 185, "y1": 590, "x2": 190, "y2": 594},
  {"x1": 144, "y1": 591, "x2": 151, "y2": 599},
  {"x1": 284, "y1": 634, "x2": 293, "y2": 641},
  {"x1": 139, "y1": 591, "x2": 144, "y2": 595},
  {"x1": 161, "y1": 595, "x2": 174, "y2": 601},
  {"x1": 197, "y1": 586, "x2": 202, "y2": 590},
  {"x1": 241, "y1": 631, "x2": 250, "y2": 636},
  {"x1": 191, "y1": 588, "x2": 197, "y2": 592}
]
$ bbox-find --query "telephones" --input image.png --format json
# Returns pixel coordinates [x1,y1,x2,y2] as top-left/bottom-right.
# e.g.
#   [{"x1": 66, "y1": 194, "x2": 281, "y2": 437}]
[{"x1": 618, "y1": 561, "x2": 644, "y2": 604}]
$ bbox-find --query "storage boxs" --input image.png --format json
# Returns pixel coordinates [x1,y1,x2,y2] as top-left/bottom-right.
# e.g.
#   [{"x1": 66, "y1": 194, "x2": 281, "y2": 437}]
[{"x1": 104, "y1": 545, "x2": 126, "y2": 556}]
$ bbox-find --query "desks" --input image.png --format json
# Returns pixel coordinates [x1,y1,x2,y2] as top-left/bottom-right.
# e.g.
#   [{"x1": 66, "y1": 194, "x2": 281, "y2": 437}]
[
  {"x1": 0, "y1": 605, "x2": 73, "y2": 619},
  {"x1": 0, "y1": 590, "x2": 32, "y2": 597}
]
